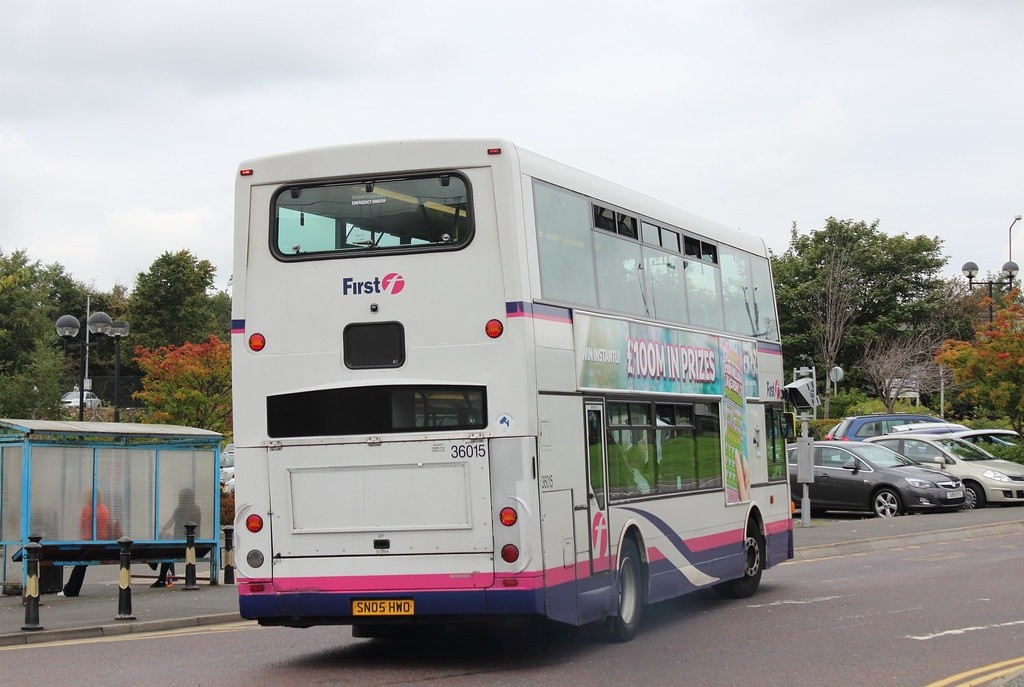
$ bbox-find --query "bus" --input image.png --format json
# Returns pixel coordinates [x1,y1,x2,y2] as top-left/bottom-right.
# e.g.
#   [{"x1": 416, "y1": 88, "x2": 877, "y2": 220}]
[{"x1": 229, "y1": 136, "x2": 797, "y2": 644}]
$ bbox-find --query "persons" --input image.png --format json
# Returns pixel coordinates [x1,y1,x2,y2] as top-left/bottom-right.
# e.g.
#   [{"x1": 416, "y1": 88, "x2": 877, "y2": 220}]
[
  {"x1": 56, "y1": 486, "x2": 110, "y2": 596},
  {"x1": 150, "y1": 488, "x2": 201, "y2": 588},
  {"x1": 734, "y1": 448, "x2": 749, "y2": 502}
]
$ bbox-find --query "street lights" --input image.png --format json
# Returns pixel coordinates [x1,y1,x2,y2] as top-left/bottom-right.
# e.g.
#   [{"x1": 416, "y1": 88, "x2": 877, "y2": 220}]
[
  {"x1": 962, "y1": 261, "x2": 1019, "y2": 336},
  {"x1": 55, "y1": 312, "x2": 111, "y2": 421},
  {"x1": 1007, "y1": 214, "x2": 1022, "y2": 262},
  {"x1": 106, "y1": 319, "x2": 130, "y2": 423}
]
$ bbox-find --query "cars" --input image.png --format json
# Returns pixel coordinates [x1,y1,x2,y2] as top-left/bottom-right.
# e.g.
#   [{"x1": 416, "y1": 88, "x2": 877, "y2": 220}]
[
  {"x1": 58, "y1": 391, "x2": 102, "y2": 409},
  {"x1": 769, "y1": 440, "x2": 965, "y2": 519},
  {"x1": 824, "y1": 414, "x2": 1023, "y2": 458},
  {"x1": 219, "y1": 444, "x2": 235, "y2": 495},
  {"x1": 847, "y1": 433, "x2": 1024, "y2": 509}
]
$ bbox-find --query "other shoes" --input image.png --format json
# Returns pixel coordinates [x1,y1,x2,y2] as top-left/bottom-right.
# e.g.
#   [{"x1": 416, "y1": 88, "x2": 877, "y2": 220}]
[
  {"x1": 150, "y1": 580, "x2": 166, "y2": 587},
  {"x1": 57, "y1": 591, "x2": 65, "y2": 596},
  {"x1": 149, "y1": 563, "x2": 158, "y2": 570}
]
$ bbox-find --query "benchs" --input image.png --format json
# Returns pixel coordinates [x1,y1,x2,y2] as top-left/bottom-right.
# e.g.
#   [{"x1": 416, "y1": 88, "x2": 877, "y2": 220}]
[{"x1": 12, "y1": 541, "x2": 215, "y2": 563}]
